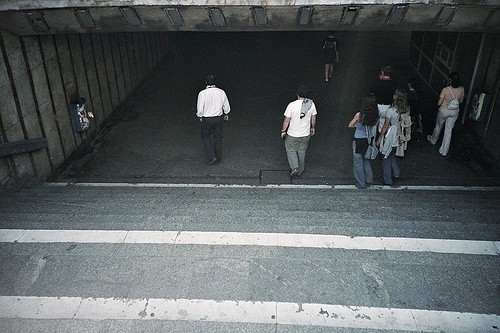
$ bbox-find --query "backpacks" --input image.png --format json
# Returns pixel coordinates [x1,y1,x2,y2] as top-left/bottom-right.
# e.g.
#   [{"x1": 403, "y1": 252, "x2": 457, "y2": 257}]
[
  {"x1": 394, "y1": 105, "x2": 411, "y2": 141},
  {"x1": 444, "y1": 87, "x2": 462, "y2": 110}
]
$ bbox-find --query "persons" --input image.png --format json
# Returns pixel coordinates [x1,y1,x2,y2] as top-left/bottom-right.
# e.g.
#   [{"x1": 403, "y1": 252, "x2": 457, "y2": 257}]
[
  {"x1": 348, "y1": 93, "x2": 379, "y2": 188},
  {"x1": 427, "y1": 72, "x2": 464, "y2": 156},
  {"x1": 376, "y1": 88, "x2": 409, "y2": 185},
  {"x1": 195, "y1": 74, "x2": 230, "y2": 165},
  {"x1": 368, "y1": 64, "x2": 397, "y2": 105},
  {"x1": 322, "y1": 34, "x2": 338, "y2": 82},
  {"x1": 281, "y1": 86, "x2": 317, "y2": 177},
  {"x1": 406, "y1": 78, "x2": 423, "y2": 134}
]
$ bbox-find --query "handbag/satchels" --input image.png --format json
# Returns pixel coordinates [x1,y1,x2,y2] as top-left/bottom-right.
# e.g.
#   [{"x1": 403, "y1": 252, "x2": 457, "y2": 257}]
[{"x1": 364, "y1": 145, "x2": 379, "y2": 160}]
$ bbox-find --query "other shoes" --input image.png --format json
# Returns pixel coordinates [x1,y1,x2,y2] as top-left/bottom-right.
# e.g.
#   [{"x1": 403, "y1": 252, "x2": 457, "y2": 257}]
[
  {"x1": 427, "y1": 135, "x2": 436, "y2": 145},
  {"x1": 208, "y1": 158, "x2": 218, "y2": 165},
  {"x1": 290, "y1": 167, "x2": 299, "y2": 176},
  {"x1": 439, "y1": 149, "x2": 446, "y2": 156}
]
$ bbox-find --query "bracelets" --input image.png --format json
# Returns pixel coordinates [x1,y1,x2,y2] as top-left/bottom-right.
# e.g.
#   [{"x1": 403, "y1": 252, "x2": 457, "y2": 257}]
[{"x1": 281, "y1": 130, "x2": 286, "y2": 132}]
